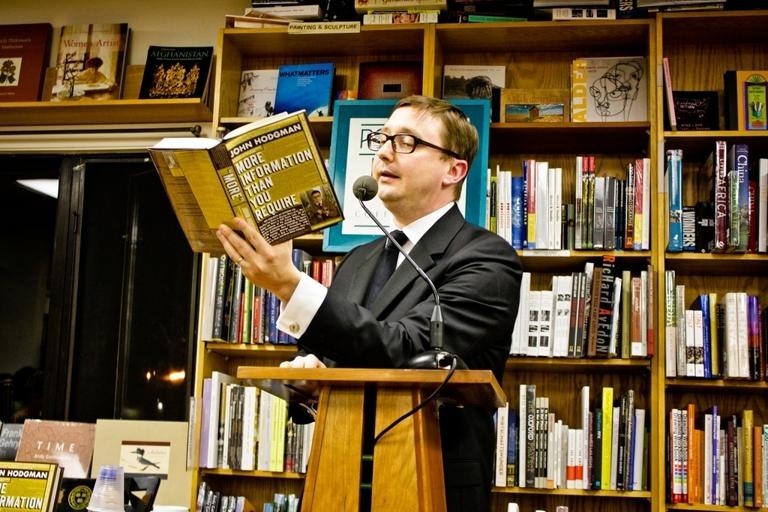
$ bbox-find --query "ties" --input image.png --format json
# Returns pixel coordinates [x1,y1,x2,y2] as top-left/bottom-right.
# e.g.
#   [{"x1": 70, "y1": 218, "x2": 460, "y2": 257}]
[
  {"x1": 364, "y1": 231, "x2": 408, "y2": 307},
  {"x1": 318, "y1": 202, "x2": 322, "y2": 207}
]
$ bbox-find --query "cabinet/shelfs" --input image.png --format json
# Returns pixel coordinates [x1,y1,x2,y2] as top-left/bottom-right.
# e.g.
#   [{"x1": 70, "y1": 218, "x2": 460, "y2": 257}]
[
  {"x1": 657, "y1": 12, "x2": 768, "y2": 512},
  {"x1": 190, "y1": 20, "x2": 658, "y2": 510},
  {"x1": 1, "y1": 62, "x2": 213, "y2": 127}
]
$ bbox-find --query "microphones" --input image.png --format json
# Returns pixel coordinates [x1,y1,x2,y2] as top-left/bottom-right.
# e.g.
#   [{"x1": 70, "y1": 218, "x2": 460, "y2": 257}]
[{"x1": 353, "y1": 174, "x2": 471, "y2": 371}]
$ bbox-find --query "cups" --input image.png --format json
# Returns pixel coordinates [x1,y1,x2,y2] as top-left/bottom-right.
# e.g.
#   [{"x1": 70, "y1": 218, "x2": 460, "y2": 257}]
[{"x1": 87, "y1": 465, "x2": 123, "y2": 512}]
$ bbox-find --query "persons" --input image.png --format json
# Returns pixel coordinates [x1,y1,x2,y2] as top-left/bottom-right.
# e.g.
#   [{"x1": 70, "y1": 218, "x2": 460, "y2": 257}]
[
  {"x1": 216, "y1": 96, "x2": 522, "y2": 512},
  {"x1": 303, "y1": 190, "x2": 338, "y2": 222}
]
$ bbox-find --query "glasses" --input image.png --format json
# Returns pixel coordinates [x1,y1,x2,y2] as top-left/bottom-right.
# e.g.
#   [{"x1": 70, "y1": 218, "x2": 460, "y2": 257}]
[{"x1": 369, "y1": 128, "x2": 462, "y2": 162}]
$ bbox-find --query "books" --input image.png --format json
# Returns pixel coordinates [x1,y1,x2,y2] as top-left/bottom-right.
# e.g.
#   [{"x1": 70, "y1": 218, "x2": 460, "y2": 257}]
[
  {"x1": 200, "y1": 246, "x2": 333, "y2": 345},
  {"x1": 137, "y1": 44, "x2": 215, "y2": 106},
  {"x1": 570, "y1": 56, "x2": 647, "y2": 122},
  {"x1": 510, "y1": 255, "x2": 654, "y2": 359},
  {"x1": 0, "y1": 419, "x2": 191, "y2": 512},
  {"x1": 147, "y1": 109, "x2": 345, "y2": 254},
  {"x1": 200, "y1": 371, "x2": 315, "y2": 474},
  {"x1": 195, "y1": 480, "x2": 300, "y2": 512},
  {"x1": 50, "y1": 20, "x2": 132, "y2": 102},
  {"x1": 664, "y1": 139, "x2": 768, "y2": 253},
  {"x1": 665, "y1": 268, "x2": 765, "y2": 382},
  {"x1": 442, "y1": 64, "x2": 506, "y2": 123},
  {"x1": 236, "y1": 63, "x2": 337, "y2": 122},
  {"x1": 661, "y1": 58, "x2": 682, "y2": 130},
  {"x1": 492, "y1": 381, "x2": 650, "y2": 492},
  {"x1": 0, "y1": 22, "x2": 53, "y2": 103},
  {"x1": 487, "y1": 155, "x2": 650, "y2": 250},
  {"x1": 223, "y1": 0, "x2": 724, "y2": 27},
  {"x1": 668, "y1": 400, "x2": 768, "y2": 507}
]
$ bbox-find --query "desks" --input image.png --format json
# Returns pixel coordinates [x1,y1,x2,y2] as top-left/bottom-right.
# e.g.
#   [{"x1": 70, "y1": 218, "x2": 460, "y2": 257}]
[{"x1": 237, "y1": 365, "x2": 506, "y2": 512}]
